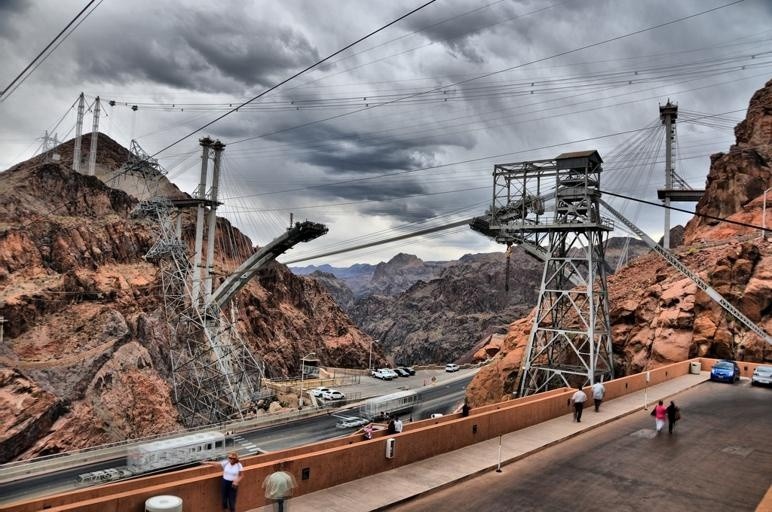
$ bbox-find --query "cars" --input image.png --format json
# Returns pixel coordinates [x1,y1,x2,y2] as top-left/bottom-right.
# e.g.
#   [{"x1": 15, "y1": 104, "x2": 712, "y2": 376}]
[
  {"x1": 335, "y1": 416, "x2": 369, "y2": 429},
  {"x1": 311, "y1": 387, "x2": 344, "y2": 401},
  {"x1": 711, "y1": 361, "x2": 772, "y2": 388},
  {"x1": 446, "y1": 364, "x2": 459, "y2": 372},
  {"x1": 72, "y1": 466, "x2": 132, "y2": 487},
  {"x1": 372, "y1": 367, "x2": 415, "y2": 381}
]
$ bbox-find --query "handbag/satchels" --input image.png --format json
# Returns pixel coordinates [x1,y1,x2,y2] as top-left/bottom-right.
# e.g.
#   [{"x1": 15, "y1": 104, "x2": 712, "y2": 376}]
[
  {"x1": 651, "y1": 405, "x2": 657, "y2": 417},
  {"x1": 675, "y1": 408, "x2": 680, "y2": 421}
]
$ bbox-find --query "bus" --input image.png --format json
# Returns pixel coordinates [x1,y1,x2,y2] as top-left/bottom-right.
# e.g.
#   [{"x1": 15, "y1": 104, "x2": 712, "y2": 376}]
[
  {"x1": 127, "y1": 431, "x2": 235, "y2": 475},
  {"x1": 360, "y1": 390, "x2": 418, "y2": 422}
]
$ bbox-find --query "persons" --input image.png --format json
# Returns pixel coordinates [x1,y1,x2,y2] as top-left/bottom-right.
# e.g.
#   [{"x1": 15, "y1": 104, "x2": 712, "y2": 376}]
[
  {"x1": 260, "y1": 462, "x2": 298, "y2": 511},
  {"x1": 662, "y1": 400, "x2": 680, "y2": 434},
  {"x1": 461, "y1": 400, "x2": 473, "y2": 417},
  {"x1": 571, "y1": 385, "x2": 589, "y2": 423},
  {"x1": 387, "y1": 420, "x2": 396, "y2": 434},
  {"x1": 394, "y1": 416, "x2": 403, "y2": 433},
  {"x1": 589, "y1": 378, "x2": 606, "y2": 412},
  {"x1": 653, "y1": 399, "x2": 667, "y2": 434},
  {"x1": 199, "y1": 451, "x2": 246, "y2": 511},
  {"x1": 361, "y1": 422, "x2": 374, "y2": 440}
]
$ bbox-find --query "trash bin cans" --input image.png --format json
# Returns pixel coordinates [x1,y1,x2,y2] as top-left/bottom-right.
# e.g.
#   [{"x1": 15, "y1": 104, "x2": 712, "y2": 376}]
[
  {"x1": 145, "y1": 495, "x2": 183, "y2": 512},
  {"x1": 691, "y1": 362, "x2": 701, "y2": 375}
]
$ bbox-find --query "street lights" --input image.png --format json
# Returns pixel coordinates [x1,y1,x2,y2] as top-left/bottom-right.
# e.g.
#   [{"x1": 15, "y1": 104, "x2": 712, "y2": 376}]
[
  {"x1": 299, "y1": 353, "x2": 317, "y2": 406},
  {"x1": 369, "y1": 340, "x2": 379, "y2": 369}
]
